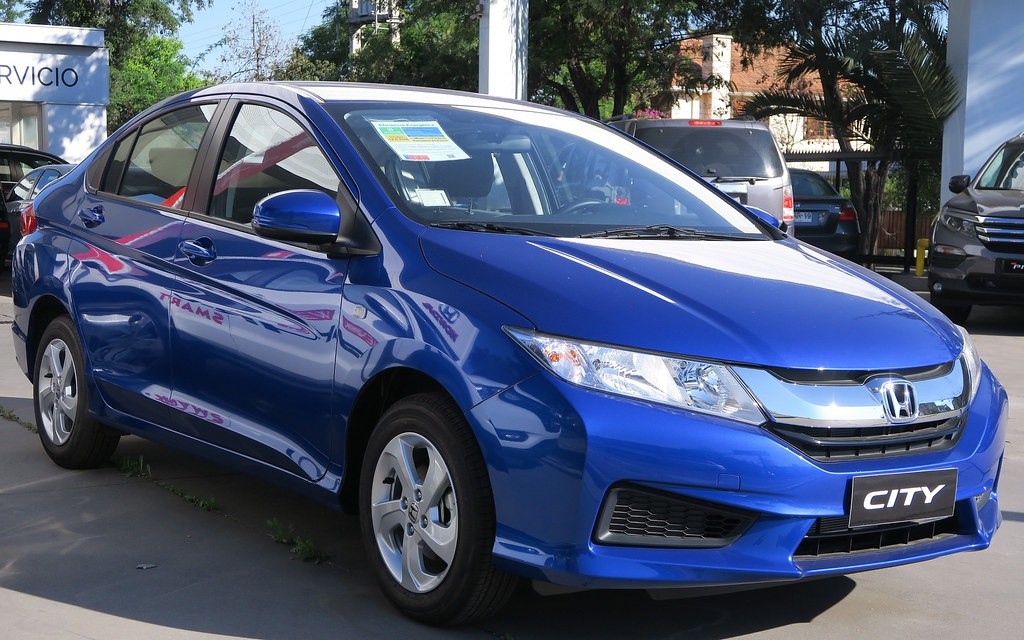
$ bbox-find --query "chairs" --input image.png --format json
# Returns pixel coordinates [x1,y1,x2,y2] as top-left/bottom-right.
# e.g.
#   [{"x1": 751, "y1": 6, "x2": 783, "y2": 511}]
[{"x1": 427, "y1": 149, "x2": 502, "y2": 219}]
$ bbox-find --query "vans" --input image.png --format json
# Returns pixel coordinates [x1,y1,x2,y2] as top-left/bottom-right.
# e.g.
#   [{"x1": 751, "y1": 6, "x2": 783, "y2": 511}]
[{"x1": 602, "y1": 116, "x2": 797, "y2": 241}]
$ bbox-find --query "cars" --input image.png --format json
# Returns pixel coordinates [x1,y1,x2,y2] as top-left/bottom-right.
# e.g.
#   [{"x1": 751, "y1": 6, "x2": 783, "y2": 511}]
[
  {"x1": 933, "y1": 126, "x2": 1024, "y2": 335},
  {"x1": 790, "y1": 165, "x2": 863, "y2": 256},
  {"x1": 0, "y1": 142, "x2": 64, "y2": 293},
  {"x1": 9, "y1": 74, "x2": 1008, "y2": 627}
]
